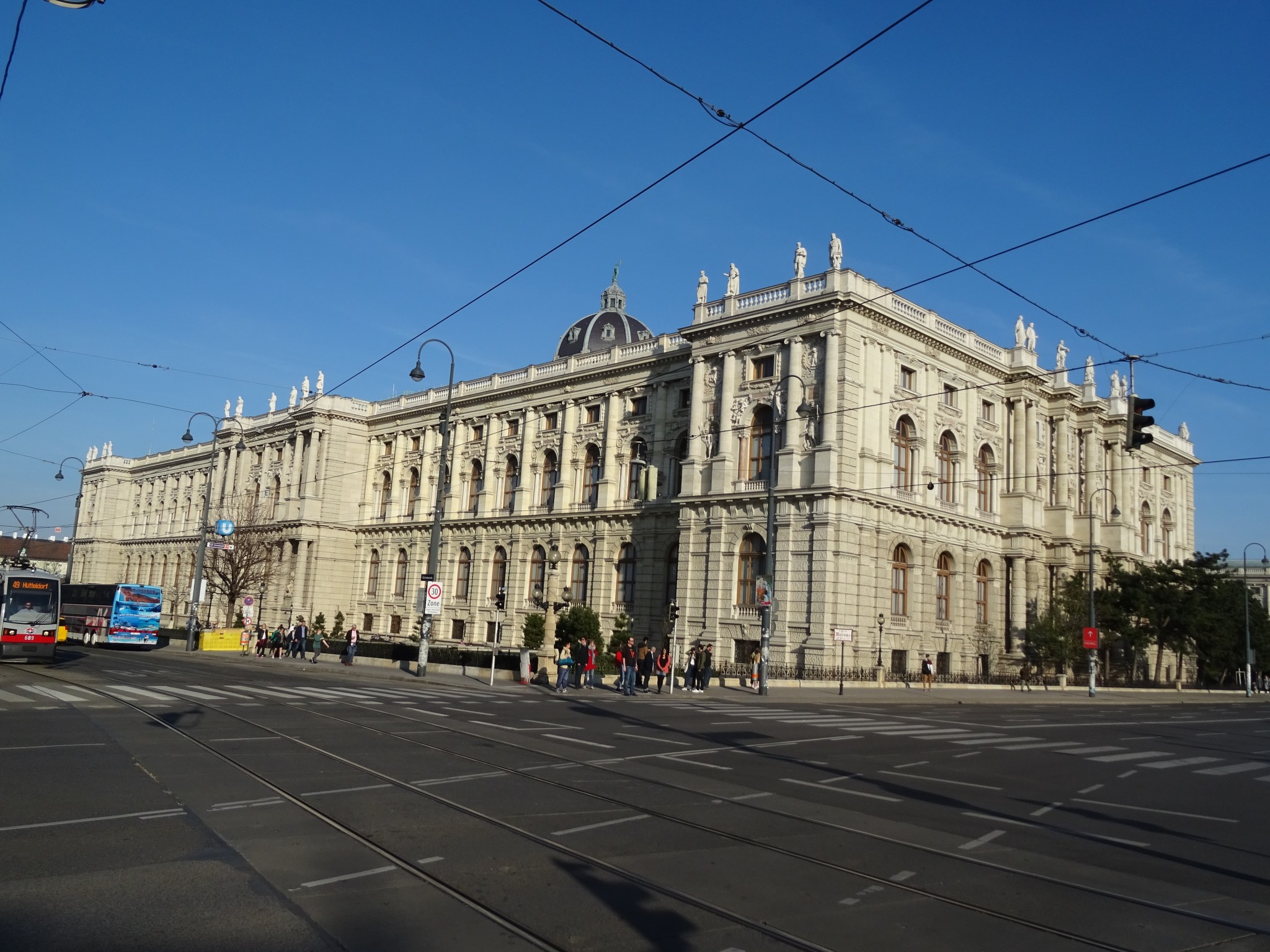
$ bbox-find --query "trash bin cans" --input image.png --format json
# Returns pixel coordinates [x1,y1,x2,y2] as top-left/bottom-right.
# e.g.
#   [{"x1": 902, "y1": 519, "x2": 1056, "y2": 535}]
[
  {"x1": 239, "y1": 631, "x2": 251, "y2": 646},
  {"x1": 751, "y1": 662, "x2": 759, "y2": 681}
]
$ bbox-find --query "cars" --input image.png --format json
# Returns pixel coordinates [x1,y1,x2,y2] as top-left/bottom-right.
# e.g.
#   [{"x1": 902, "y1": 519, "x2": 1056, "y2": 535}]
[{"x1": 57, "y1": 617, "x2": 68, "y2": 641}]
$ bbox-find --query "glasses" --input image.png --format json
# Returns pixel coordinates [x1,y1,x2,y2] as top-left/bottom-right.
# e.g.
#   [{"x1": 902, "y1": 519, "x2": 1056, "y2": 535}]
[
  {"x1": 580, "y1": 638, "x2": 585, "y2": 641},
  {"x1": 629, "y1": 640, "x2": 634, "y2": 642}
]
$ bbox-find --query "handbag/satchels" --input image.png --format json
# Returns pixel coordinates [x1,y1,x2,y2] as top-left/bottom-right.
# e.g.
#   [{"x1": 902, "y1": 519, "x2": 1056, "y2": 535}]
[
  {"x1": 558, "y1": 651, "x2": 574, "y2": 665},
  {"x1": 684, "y1": 662, "x2": 688, "y2": 673}
]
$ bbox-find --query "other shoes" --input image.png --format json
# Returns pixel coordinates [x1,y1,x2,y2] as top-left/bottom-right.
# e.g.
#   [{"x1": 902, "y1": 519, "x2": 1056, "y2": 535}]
[
  {"x1": 616, "y1": 688, "x2": 623, "y2": 691},
  {"x1": 624, "y1": 693, "x2": 630, "y2": 696},
  {"x1": 751, "y1": 685, "x2": 757, "y2": 689},
  {"x1": 1028, "y1": 689, "x2": 1031, "y2": 692},
  {"x1": 348, "y1": 664, "x2": 353, "y2": 666},
  {"x1": 345, "y1": 661, "x2": 348, "y2": 665},
  {"x1": 1020, "y1": 689, "x2": 1025, "y2": 692},
  {"x1": 590, "y1": 686, "x2": 594, "y2": 689},
  {"x1": 681, "y1": 686, "x2": 711, "y2": 692},
  {"x1": 923, "y1": 689, "x2": 927, "y2": 691},
  {"x1": 643, "y1": 690, "x2": 652, "y2": 693},
  {"x1": 630, "y1": 693, "x2": 638, "y2": 696},
  {"x1": 657, "y1": 690, "x2": 660, "y2": 693},
  {"x1": 929, "y1": 688, "x2": 932, "y2": 691},
  {"x1": 584, "y1": 686, "x2": 586, "y2": 688},
  {"x1": 255, "y1": 653, "x2": 318, "y2": 666}
]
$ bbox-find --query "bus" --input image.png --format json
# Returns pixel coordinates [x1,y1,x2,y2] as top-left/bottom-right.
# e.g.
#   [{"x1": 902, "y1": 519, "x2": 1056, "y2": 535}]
[
  {"x1": 0, "y1": 556, "x2": 61, "y2": 663},
  {"x1": 59, "y1": 583, "x2": 162, "y2": 648}
]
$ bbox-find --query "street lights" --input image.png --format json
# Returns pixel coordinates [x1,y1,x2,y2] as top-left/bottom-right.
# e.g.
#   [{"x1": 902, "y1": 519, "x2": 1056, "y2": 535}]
[
  {"x1": 252, "y1": 582, "x2": 266, "y2": 633},
  {"x1": 630, "y1": 614, "x2": 635, "y2": 634},
  {"x1": 874, "y1": 612, "x2": 885, "y2": 669},
  {"x1": 409, "y1": 339, "x2": 455, "y2": 675},
  {"x1": 1089, "y1": 488, "x2": 1121, "y2": 697},
  {"x1": 1057, "y1": 633, "x2": 1065, "y2": 674},
  {"x1": 287, "y1": 605, "x2": 293, "y2": 629},
  {"x1": 181, "y1": 412, "x2": 246, "y2": 651},
  {"x1": 54, "y1": 457, "x2": 84, "y2": 584},
  {"x1": 759, "y1": 375, "x2": 810, "y2": 696},
  {"x1": 1244, "y1": 543, "x2": 1269, "y2": 698},
  {"x1": 204, "y1": 583, "x2": 216, "y2": 629},
  {"x1": 532, "y1": 544, "x2": 574, "y2": 685}
]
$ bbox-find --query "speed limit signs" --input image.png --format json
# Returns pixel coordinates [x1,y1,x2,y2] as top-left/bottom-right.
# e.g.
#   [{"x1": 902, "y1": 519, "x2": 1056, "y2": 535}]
[{"x1": 428, "y1": 584, "x2": 441, "y2": 599}]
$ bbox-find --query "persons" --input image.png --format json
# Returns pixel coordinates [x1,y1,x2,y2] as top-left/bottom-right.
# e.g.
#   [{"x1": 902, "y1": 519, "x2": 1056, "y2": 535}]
[
  {"x1": 556, "y1": 641, "x2": 571, "y2": 693},
  {"x1": 922, "y1": 654, "x2": 933, "y2": 692},
  {"x1": 460, "y1": 640, "x2": 472, "y2": 646},
  {"x1": 210, "y1": 623, "x2": 218, "y2": 628},
  {"x1": 682, "y1": 643, "x2": 713, "y2": 693},
  {"x1": 345, "y1": 624, "x2": 360, "y2": 666},
  {"x1": 622, "y1": 636, "x2": 638, "y2": 696},
  {"x1": 642, "y1": 646, "x2": 656, "y2": 693},
  {"x1": 1020, "y1": 664, "x2": 1032, "y2": 692},
  {"x1": 751, "y1": 648, "x2": 760, "y2": 690},
  {"x1": 616, "y1": 644, "x2": 624, "y2": 693},
  {"x1": 655, "y1": 647, "x2": 671, "y2": 694},
  {"x1": 249, "y1": 620, "x2": 330, "y2": 665},
  {"x1": 121, "y1": 586, "x2": 160, "y2": 603},
  {"x1": 1256, "y1": 675, "x2": 1270, "y2": 693},
  {"x1": 19, "y1": 602, "x2": 38, "y2": 613},
  {"x1": 574, "y1": 636, "x2": 598, "y2": 690},
  {"x1": 636, "y1": 637, "x2": 650, "y2": 686},
  {"x1": 371, "y1": 633, "x2": 392, "y2": 642}
]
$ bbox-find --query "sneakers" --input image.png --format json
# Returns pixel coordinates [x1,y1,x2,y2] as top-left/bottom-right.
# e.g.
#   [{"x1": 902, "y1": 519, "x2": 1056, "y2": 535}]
[
  {"x1": 563, "y1": 690, "x2": 569, "y2": 692},
  {"x1": 556, "y1": 689, "x2": 561, "y2": 692}
]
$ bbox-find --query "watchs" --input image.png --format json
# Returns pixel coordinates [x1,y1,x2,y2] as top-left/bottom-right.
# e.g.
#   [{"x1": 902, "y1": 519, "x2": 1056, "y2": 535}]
[{"x1": 636, "y1": 665, "x2": 638, "y2": 666}]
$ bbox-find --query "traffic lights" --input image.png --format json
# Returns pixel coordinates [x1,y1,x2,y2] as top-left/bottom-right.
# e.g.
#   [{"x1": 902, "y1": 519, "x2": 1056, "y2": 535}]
[
  {"x1": 495, "y1": 594, "x2": 505, "y2": 609},
  {"x1": 1125, "y1": 396, "x2": 1155, "y2": 452},
  {"x1": 669, "y1": 605, "x2": 679, "y2": 621}
]
